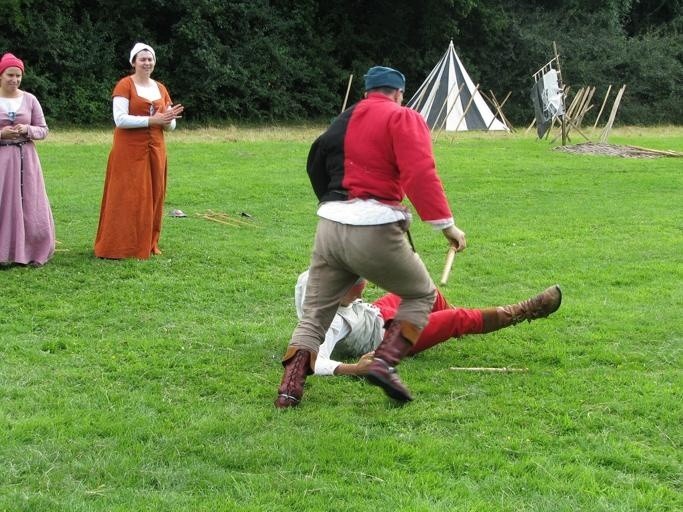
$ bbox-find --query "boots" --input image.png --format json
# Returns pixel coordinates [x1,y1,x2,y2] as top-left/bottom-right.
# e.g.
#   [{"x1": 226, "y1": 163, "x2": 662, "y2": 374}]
[
  {"x1": 364, "y1": 318, "x2": 422, "y2": 400},
  {"x1": 480, "y1": 284, "x2": 562, "y2": 333},
  {"x1": 273, "y1": 345, "x2": 317, "y2": 408}
]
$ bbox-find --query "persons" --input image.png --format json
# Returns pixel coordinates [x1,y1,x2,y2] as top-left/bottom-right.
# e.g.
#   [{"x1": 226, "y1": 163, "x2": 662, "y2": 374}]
[
  {"x1": 0, "y1": 50, "x2": 56, "y2": 268},
  {"x1": 93, "y1": 42, "x2": 185, "y2": 261},
  {"x1": 275, "y1": 63, "x2": 466, "y2": 409},
  {"x1": 294, "y1": 267, "x2": 562, "y2": 376}
]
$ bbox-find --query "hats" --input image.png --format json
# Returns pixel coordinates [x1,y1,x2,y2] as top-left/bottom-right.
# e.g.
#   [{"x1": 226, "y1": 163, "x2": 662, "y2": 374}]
[
  {"x1": 364, "y1": 66, "x2": 406, "y2": 91},
  {"x1": 0, "y1": 52, "x2": 24, "y2": 74},
  {"x1": 129, "y1": 42, "x2": 156, "y2": 67}
]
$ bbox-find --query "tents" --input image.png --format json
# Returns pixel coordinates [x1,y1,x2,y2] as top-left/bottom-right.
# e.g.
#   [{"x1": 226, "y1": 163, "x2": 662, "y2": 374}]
[{"x1": 403, "y1": 41, "x2": 512, "y2": 131}]
